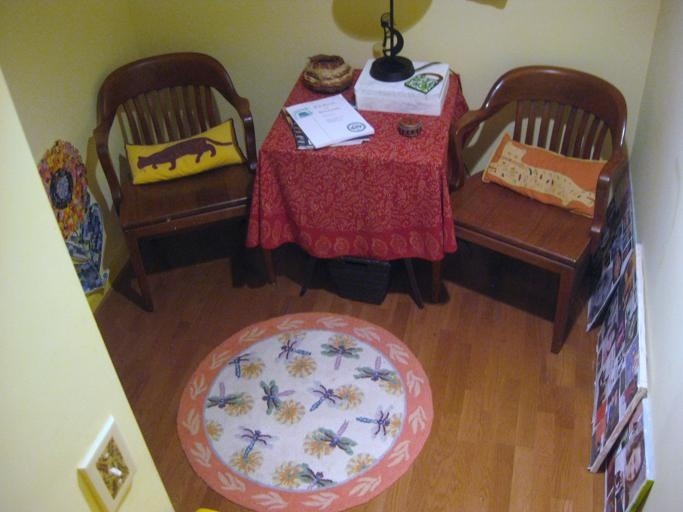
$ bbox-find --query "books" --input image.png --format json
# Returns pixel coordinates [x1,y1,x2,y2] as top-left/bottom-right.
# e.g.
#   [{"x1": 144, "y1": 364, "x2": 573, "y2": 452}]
[{"x1": 279, "y1": 93, "x2": 375, "y2": 150}]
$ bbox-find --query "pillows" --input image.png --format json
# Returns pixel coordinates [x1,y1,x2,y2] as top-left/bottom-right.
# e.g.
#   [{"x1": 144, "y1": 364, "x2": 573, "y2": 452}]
[
  {"x1": 123, "y1": 118, "x2": 247, "y2": 184},
  {"x1": 482, "y1": 132, "x2": 617, "y2": 223}
]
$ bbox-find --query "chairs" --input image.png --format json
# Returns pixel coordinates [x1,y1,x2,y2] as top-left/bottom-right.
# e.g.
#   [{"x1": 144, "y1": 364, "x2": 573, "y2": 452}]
[
  {"x1": 441, "y1": 64, "x2": 629, "y2": 353},
  {"x1": 93, "y1": 50, "x2": 283, "y2": 314}
]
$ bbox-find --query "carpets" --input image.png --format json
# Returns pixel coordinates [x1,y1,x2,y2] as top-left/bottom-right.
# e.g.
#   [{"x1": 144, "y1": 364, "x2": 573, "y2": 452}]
[{"x1": 176, "y1": 310, "x2": 433, "y2": 512}]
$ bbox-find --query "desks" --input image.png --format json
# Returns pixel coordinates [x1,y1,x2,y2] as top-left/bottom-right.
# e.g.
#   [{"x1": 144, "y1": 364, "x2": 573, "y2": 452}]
[{"x1": 248, "y1": 56, "x2": 470, "y2": 307}]
